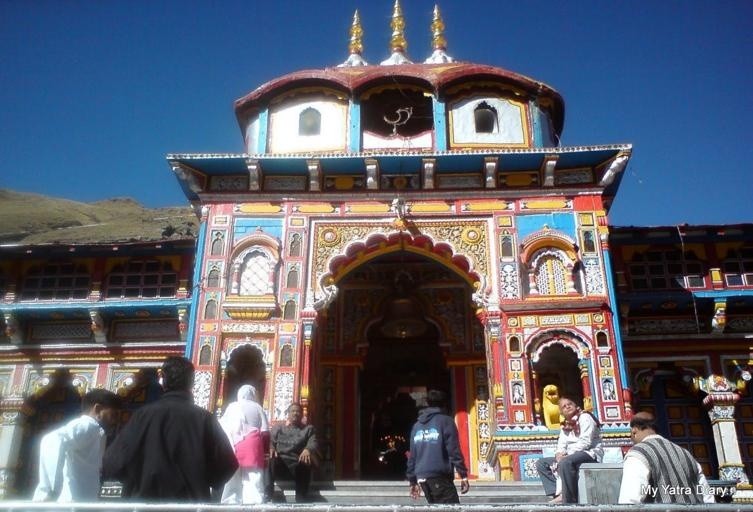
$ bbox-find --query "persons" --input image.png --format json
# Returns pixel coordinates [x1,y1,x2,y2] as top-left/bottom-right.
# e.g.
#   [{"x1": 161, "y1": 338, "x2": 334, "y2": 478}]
[
  {"x1": 618, "y1": 410, "x2": 717, "y2": 503},
  {"x1": 534, "y1": 397, "x2": 604, "y2": 503},
  {"x1": 218, "y1": 384, "x2": 271, "y2": 504},
  {"x1": 406, "y1": 389, "x2": 469, "y2": 504},
  {"x1": 30, "y1": 387, "x2": 123, "y2": 500},
  {"x1": 103, "y1": 354, "x2": 239, "y2": 503},
  {"x1": 269, "y1": 402, "x2": 320, "y2": 503}
]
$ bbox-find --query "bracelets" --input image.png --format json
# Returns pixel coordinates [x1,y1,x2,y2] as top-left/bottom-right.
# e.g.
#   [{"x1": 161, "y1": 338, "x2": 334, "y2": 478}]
[{"x1": 462, "y1": 478, "x2": 467, "y2": 481}]
